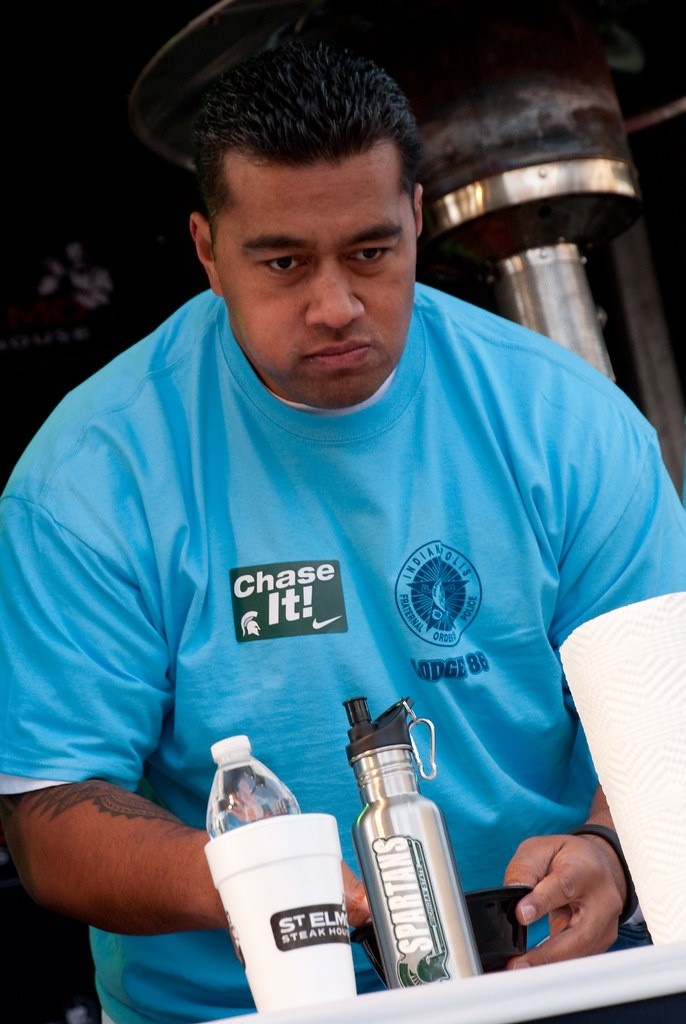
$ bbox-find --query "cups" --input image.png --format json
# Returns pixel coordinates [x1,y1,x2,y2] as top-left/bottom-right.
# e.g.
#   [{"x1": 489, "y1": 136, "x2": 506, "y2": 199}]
[{"x1": 203, "y1": 812, "x2": 356, "y2": 1015}]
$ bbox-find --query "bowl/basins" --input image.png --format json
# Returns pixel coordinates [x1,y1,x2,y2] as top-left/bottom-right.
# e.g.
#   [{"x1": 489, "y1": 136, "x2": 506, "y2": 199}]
[{"x1": 350, "y1": 880, "x2": 532, "y2": 991}]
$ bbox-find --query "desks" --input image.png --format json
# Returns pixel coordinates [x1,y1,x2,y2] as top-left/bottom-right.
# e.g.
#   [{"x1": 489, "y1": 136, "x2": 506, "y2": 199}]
[{"x1": 198, "y1": 938, "x2": 686, "y2": 1024}]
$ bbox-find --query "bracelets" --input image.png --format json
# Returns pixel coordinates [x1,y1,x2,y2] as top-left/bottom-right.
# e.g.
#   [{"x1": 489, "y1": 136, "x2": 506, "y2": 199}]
[{"x1": 569, "y1": 824, "x2": 639, "y2": 926}]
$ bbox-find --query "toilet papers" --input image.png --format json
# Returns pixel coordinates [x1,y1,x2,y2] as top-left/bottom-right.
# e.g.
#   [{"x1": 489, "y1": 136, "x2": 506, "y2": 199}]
[{"x1": 558, "y1": 590, "x2": 686, "y2": 946}]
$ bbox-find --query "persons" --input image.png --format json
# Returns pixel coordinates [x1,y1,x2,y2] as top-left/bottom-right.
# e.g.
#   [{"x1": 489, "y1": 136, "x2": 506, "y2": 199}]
[{"x1": 0, "y1": 23, "x2": 684, "y2": 1024}]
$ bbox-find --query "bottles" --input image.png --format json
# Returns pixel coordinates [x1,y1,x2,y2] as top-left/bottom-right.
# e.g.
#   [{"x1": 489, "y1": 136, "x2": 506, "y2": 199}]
[{"x1": 205, "y1": 735, "x2": 301, "y2": 840}]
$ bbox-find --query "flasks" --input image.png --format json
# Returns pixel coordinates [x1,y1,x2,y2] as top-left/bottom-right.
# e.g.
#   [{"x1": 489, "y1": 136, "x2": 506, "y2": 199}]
[{"x1": 344, "y1": 698, "x2": 481, "y2": 990}]
